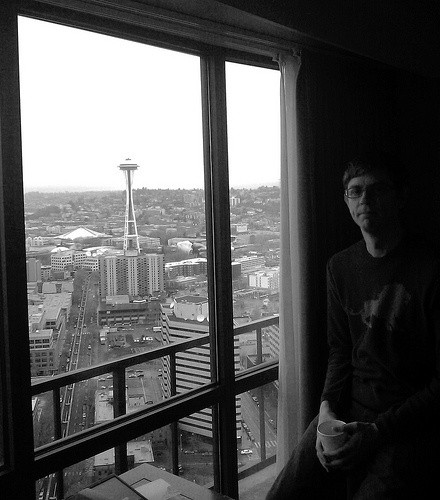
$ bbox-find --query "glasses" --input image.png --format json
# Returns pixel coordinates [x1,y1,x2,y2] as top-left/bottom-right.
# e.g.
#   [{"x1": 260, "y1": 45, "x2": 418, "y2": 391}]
[{"x1": 344, "y1": 186, "x2": 388, "y2": 199}]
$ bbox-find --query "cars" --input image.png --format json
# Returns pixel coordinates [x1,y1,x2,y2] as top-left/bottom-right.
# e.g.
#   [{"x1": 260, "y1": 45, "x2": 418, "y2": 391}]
[{"x1": 35, "y1": 271, "x2": 278, "y2": 500}]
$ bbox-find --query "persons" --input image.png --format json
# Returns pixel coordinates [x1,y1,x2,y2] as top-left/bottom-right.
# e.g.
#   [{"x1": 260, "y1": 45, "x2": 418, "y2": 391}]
[{"x1": 265, "y1": 155, "x2": 440, "y2": 500}]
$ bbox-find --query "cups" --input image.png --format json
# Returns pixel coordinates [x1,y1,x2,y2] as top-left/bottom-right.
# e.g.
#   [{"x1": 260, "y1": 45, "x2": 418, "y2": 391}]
[{"x1": 317, "y1": 420, "x2": 347, "y2": 450}]
